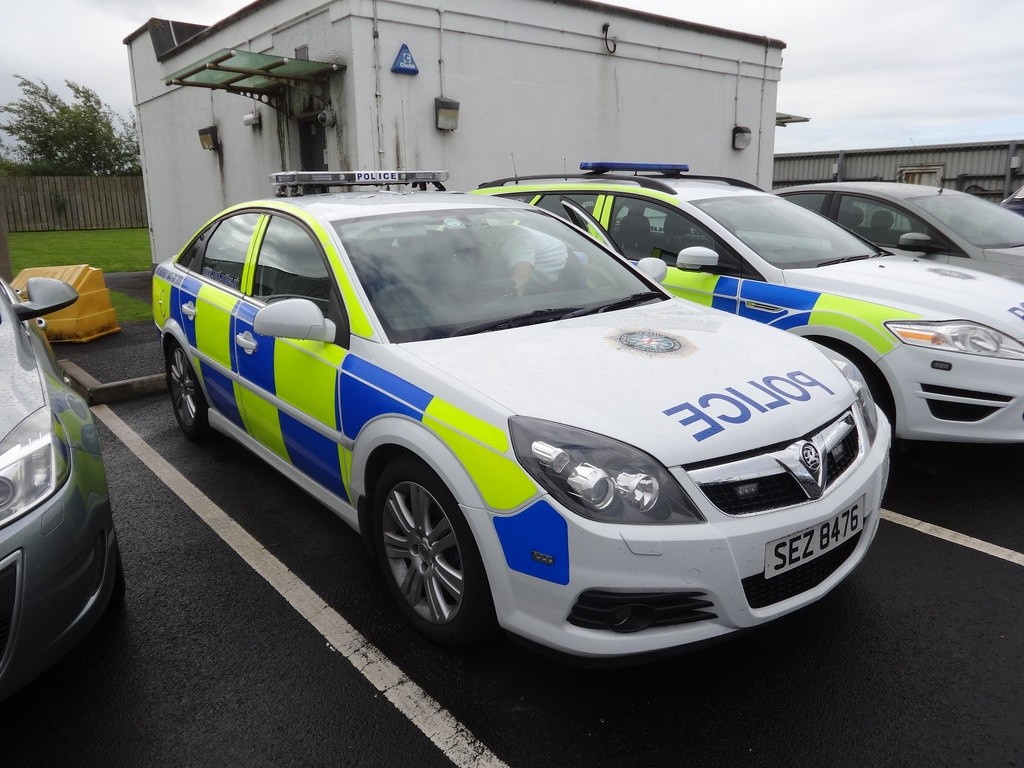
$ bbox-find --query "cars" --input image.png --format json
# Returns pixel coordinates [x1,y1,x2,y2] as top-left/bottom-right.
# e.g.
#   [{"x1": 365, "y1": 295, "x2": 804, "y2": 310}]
[
  {"x1": 998, "y1": 181, "x2": 1024, "y2": 216},
  {"x1": 767, "y1": 181, "x2": 1024, "y2": 286},
  {"x1": 0, "y1": 277, "x2": 126, "y2": 705},
  {"x1": 464, "y1": 163, "x2": 1024, "y2": 455},
  {"x1": 152, "y1": 169, "x2": 894, "y2": 667}
]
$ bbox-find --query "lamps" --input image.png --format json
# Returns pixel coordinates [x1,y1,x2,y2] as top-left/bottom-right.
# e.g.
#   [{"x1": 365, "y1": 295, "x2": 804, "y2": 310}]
[
  {"x1": 198, "y1": 126, "x2": 218, "y2": 151},
  {"x1": 434, "y1": 98, "x2": 460, "y2": 131},
  {"x1": 732, "y1": 126, "x2": 751, "y2": 150}
]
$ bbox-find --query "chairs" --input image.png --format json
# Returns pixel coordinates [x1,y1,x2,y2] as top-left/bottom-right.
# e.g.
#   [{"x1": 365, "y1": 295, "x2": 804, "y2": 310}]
[
  {"x1": 620, "y1": 215, "x2": 651, "y2": 257},
  {"x1": 838, "y1": 206, "x2": 863, "y2": 235},
  {"x1": 663, "y1": 212, "x2": 694, "y2": 261},
  {"x1": 870, "y1": 211, "x2": 898, "y2": 244}
]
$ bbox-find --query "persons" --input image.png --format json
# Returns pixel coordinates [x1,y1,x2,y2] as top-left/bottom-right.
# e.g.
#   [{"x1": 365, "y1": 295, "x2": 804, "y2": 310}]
[{"x1": 443, "y1": 217, "x2": 584, "y2": 299}]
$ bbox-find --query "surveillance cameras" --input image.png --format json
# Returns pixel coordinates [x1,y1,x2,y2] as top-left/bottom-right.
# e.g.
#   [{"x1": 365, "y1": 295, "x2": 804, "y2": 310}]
[{"x1": 318, "y1": 111, "x2": 335, "y2": 128}]
